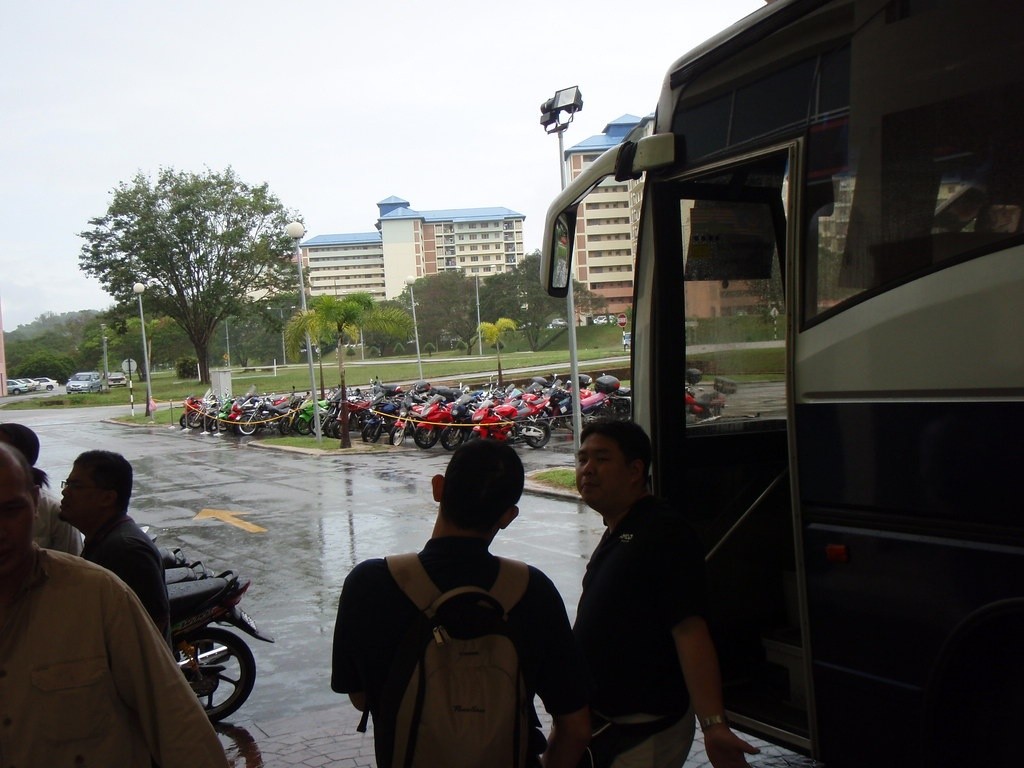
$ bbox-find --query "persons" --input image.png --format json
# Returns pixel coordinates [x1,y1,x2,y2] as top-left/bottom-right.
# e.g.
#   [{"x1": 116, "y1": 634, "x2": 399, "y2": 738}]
[
  {"x1": 0, "y1": 441, "x2": 239, "y2": 768},
  {"x1": 573, "y1": 418, "x2": 760, "y2": 768},
  {"x1": 0, "y1": 424, "x2": 83, "y2": 556},
  {"x1": 58, "y1": 451, "x2": 173, "y2": 655},
  {"x1": 331, "y1": 440, "x2": 590, "y2": 768}
]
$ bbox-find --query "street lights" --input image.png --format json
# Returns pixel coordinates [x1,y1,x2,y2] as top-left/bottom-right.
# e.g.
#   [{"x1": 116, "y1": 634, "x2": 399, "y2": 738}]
[
  {"x1": 407, "y1": 276, "x2": 428, "y2": 379},
  {"x1": 288, "y1": 221, "x2": 325, "y2": 444},
  {"x1": 540, "y1": 85, "x2": 588, "y2": 514},
  {"x1": 134, "y1": 282, "x2": 158, "y2": 424},
  {"x1": 100, "y1": 323, "x2": 110, "y2": 391},
  {"x1": 216, "y1": 316, "x2": 239, "y2": 367},
  {"x1": 267, "y1": 306, "x2": 296, "y2": 365}
]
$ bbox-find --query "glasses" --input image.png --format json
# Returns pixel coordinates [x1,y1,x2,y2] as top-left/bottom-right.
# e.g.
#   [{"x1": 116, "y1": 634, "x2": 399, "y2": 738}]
[{"x1": 61, "y1": 481, "x2": 110, "y2": 493}]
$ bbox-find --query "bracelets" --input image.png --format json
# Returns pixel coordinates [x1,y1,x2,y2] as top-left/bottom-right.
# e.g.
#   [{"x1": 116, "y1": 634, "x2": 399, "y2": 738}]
[{"x1": 700, "y1": 715, "x2": 728, "y2": 732}]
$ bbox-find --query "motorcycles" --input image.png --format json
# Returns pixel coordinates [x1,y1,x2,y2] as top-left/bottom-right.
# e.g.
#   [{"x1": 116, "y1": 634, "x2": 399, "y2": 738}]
[
  {"x1": 141, "y1": 522, "x2": 275, "y2": 723},
  {"x1": 179, "y1": 368, "x2": 738, "y2": 453}
]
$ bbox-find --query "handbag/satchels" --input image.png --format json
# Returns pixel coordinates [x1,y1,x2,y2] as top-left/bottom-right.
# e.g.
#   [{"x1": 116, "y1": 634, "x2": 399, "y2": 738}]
[{"x1": 579, "y1": 694, "x2": 690, "y2": 768}]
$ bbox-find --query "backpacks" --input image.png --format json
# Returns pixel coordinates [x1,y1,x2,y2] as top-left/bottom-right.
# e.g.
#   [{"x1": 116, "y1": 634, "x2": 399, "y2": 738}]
[{"x1": 384, "y1": 554, "x2": 530, "y2": 768}]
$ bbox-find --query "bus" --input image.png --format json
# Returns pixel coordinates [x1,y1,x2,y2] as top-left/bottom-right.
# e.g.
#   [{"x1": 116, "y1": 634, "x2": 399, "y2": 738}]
[{"x1": 540, "y1": 0, "x2": 1023, "y2": 768}]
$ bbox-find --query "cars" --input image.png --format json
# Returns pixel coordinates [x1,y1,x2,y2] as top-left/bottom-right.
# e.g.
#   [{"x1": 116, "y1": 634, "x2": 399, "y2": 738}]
[
  {"x1": 32, "y1": 377, "x2": 60, "y2": 392},
  {"x1": 66, "y1": 372, "x2": 103, "y2": 395},
  {"x1": 5, "y1": 378, "x2": 40, "y2": 395},
  {"x1": 108, "y1": 371, "x2": 128, "y2": 387},
  {"x1": 519, "y1": 322, "x2": 540, "y2": 329},
  {"x1": 593, "y1": 315, "x2": 615, "y2": 325},
  {"x1": 547, "y1": 318, "x2": 568, "y2": 328},
  {"x1": 622, "y1": 332, "x2": 631, "y2": 351}
]
